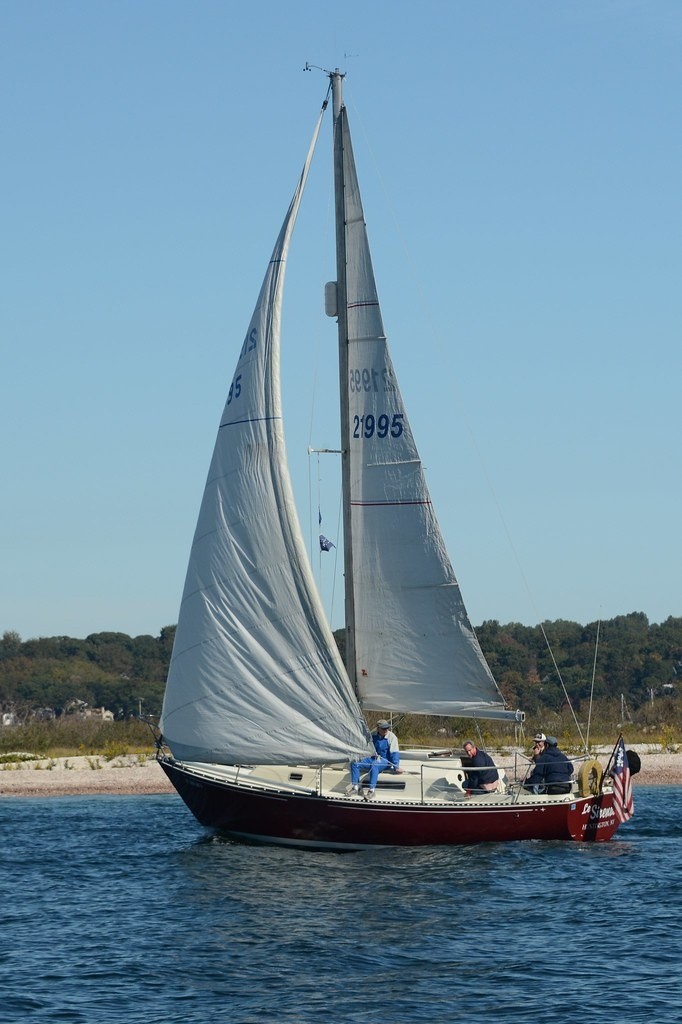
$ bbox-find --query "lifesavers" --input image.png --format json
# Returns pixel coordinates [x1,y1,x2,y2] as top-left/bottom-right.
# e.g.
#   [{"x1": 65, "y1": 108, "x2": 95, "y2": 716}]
[{"x1": 579, "y1": 758, "x2": 603, "y2": 798}]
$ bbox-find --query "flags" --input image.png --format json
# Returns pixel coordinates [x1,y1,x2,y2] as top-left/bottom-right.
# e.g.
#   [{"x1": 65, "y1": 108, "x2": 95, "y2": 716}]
[{"x1": 606, "y1": 738, "x2": 634, "y2": 824}]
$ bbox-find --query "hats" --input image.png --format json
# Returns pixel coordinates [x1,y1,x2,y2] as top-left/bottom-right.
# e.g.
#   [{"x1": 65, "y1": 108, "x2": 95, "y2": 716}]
[
  {"x1": 376, "y1": 719, "x2": 389, "y2": 729},
  {"x1": 532, "y1": 733, "x2": 546, "y2": 741},
  {"x1": 546, "y1": 736, "x2": 557, "y2": 745}
]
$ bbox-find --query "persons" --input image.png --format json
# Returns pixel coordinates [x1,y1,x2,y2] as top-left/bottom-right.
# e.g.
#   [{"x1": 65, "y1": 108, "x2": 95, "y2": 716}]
[
  {"x1": 525, "y1": 736, "x2": 574, "y2": 795},
  {"x1": 529, "y1": 733, "x2": 547, "y2": 764},
  {"x1": 463, "y1": 741, "x2": 499, "y2": 800},
  {"x1": 346, "y1": 719, "x2": 402, "y2": 800}
]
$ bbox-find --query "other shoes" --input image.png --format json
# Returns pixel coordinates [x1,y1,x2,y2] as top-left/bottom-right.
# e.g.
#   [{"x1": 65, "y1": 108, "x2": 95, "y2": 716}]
[
  {"x1": 349, "y1": 788, "x2": 360, "y2": 795},
  {"x1": 365, "y1": 790, "x2": 374, "y2": 799}
]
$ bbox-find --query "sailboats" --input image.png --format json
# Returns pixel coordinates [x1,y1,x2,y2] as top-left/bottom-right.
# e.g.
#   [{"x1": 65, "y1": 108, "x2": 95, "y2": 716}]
[{"x1": 151, "y1": 62, "x2": 645, "y2": 857}]
